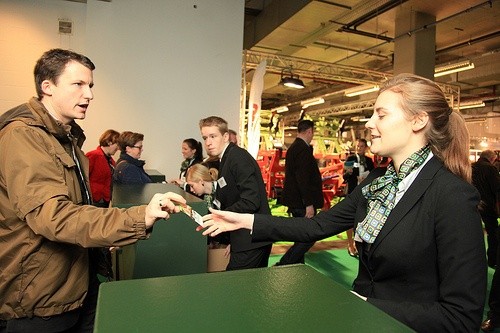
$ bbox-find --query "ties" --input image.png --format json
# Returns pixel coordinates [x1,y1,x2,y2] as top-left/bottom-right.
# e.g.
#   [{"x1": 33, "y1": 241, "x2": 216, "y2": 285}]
[
  {"x1": 180, "y1": 156, "x2": 196, "y2": 174},
  {"x1": 203, "y1": 181, "x2": 215, "y2": 211},
  {"x1": 355, "y1": 142, "x2": 432, "y2": 244}
]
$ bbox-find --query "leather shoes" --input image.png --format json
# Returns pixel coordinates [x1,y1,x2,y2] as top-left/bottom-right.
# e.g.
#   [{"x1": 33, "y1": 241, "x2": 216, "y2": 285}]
[{"x1": 482, "y1": 318, "x2": 495, "y2": 332}]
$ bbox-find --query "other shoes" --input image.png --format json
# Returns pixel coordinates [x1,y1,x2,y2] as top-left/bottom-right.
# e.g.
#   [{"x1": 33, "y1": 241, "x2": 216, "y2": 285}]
[
  {"x1": 347, "y1": 248, "x2": 357, "y2": 257},
  {"x1": 356, "y1": 251, "x2": 360, "y2": 255}
]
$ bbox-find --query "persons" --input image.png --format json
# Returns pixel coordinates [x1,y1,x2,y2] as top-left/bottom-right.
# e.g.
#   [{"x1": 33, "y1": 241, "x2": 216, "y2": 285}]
[
  {"x1": 342, "y1": 139, "x2": 375, "y2": 257},
  {"x1": 113, "y1": 131, "x2": 155, "y2": 184},
  {"x1": 85, "y1": 129, "x2": 120, "y2": 208},
  {"x1": 195, "y1": 72, "x2": 488, "y2": 333},
  {"x1": 471, "y1": 150, "x2": 500, "y2": 333},
  {"x1": 276, "y1": 120, "x2": 324, "y2": 266},
  {"x1": 0, "y1": 48, "x2": 187, "y2": 333},
  {"x1": 170, "y1": 116, "x2": 273, "y2": 272}
]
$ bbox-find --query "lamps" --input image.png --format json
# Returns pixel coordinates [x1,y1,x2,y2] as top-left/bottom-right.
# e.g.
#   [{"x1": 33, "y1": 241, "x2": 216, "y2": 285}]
[
  {"x1": 433, "y1": 60, "x2": 475, "y2": 77},
  {"x1": 345, "y1": 85, "x2": 380, "y2": 98},
  {"x1": 279, "y1": 77, "x2": 306, "y2": 89},
  {"x1": 301, "y1": 98, "x2": 325, "y2": 109},
  {"x1": 453, "y1": 101, "x2": 485, "y2": 110}
]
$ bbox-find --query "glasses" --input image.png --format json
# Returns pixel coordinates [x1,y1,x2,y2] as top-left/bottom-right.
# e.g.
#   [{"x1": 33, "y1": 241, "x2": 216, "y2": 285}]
[{"x1": 130, "y1": 144, "x2": 142, "y2": 150}]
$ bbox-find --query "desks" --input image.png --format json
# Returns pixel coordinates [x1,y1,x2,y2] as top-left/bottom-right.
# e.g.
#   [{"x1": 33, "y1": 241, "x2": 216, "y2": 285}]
[
  {"x1": 93, "y1": 262, "x2": 418, "y2": 333},
  {"x1": 112, "y1": 183, "x2": 207, "y2": 281},
  {"x1": 143, "y1": 169, "x2": 168, "y2": 184}
]
